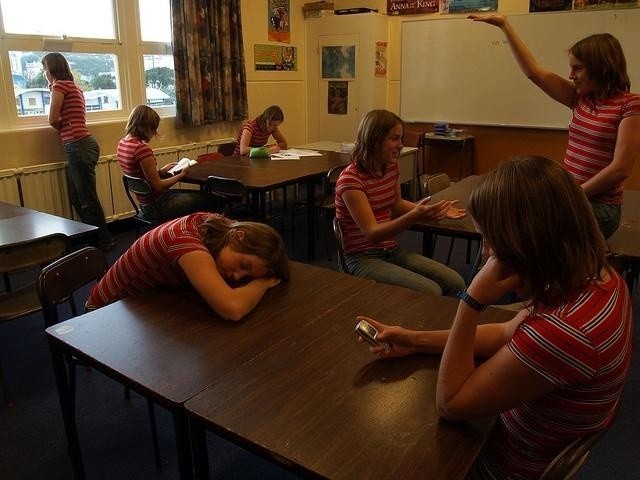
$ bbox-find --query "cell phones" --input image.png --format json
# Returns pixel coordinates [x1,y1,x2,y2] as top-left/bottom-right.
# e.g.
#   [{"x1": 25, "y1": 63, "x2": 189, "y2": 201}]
[{"x1": 354, "y1": 319, "x2": 392, "y2": 356}]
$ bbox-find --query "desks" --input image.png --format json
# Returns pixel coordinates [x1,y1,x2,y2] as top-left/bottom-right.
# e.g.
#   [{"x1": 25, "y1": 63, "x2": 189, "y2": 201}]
[
  {"x1": 45, "y1": 260, "x2": 376, "y2": 480},
  {"x1": 163, "y1": 141, "x2": 418, "y2": 225},
  {"x1": 184, "y1": 283, "x2": 518, "y2": 480},
  {"x1": 423, "y1": 134, "x2": 475, "y2": 183},
  {"x1": 409, "y1": 175, "x2": 640, "y2": 274},
  {"x1": 0, "y1": 201, "x2": 98, "y2": 292}
]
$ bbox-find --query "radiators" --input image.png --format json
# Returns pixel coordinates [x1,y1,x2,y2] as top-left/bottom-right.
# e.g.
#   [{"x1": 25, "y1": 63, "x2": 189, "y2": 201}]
[{"x1": 0, "y1": 137, "x2": 235, "y2": 227}]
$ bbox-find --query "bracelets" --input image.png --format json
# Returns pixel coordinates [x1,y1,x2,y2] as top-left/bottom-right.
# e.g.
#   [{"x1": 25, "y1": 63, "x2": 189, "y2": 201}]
[{"x1": 457, "y1": 289, "x2": 488, "y2": 312}]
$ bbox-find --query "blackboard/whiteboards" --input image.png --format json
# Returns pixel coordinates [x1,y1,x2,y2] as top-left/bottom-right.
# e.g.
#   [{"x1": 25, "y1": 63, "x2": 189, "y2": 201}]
[{"x1": 399, "y1": 7, "x2": 640, "y2": 130}]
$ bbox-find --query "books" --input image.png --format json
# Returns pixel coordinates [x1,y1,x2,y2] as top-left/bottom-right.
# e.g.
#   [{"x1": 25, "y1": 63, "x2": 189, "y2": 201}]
[
  {"x1": 166, "y1": 157, "x2": 198, "y2": 176},
  {"x1": 270, "y1": 156, "x2": 300, "y2": 161}
]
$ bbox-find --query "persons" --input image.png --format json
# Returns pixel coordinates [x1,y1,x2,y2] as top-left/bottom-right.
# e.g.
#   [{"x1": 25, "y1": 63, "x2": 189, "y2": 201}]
[
  {"x1": 334, "y1": 109, "x2": 466, "y2": 298},
  {"x1": 328, "y1": 90, "x2": 348, "y2": 114},
  {"x1": 232, "y1": 105, "x2": 287, "y2": 156},
  {"x1": 271, "y1": 10, "x2": 289, "y2": 33},
  {"x1": 117, "y1": 104, "x2": 226, "y2": 220},
  {"x1": 356, "y1": 153, "x2": 633, "y2": 480},
  {"x1": 42, "y1": 52, "x2": 116, "y2": 250},
  {"x1": 466, "y1": 13, "x2": 640, "y2": 240},
  {"x1": 83, "y1": 212, "x2": 285, "y2": 322},
  {"x1": 282, "y1": 47, "x2": 294, "y2": 71}
]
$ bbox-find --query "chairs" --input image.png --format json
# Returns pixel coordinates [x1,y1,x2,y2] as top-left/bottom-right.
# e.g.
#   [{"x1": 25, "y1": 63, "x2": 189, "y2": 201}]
[
  {"x1": 123, "y1": 174, "x2": 164, "y2": 238},
  {"x1": 332, "y1": 216, "x2": 349, "y2": 274},
  {"x1": 539, "y1": 431, "x2": 609, "y2": 480},
  {"x1": 198, "y1": 152, "x2": 224, "y2": 163},
  {"x1": 37, "y1": 247, "x2": 162, "y2": 480},
  {"x1": 0, "y1": 233, "x2": 77, "y2": 402},
  {"x1": 425, "y1": 173, "x2": 456, "y2": 266},
  {"x1": 207, "y1": 176, "x2": 251, "y2": 218},
  {"x1": 219, "y1": 142, "x2": 237, "y2": 157},
  {"x1": 291, "y1": 164, "x2": 346, "y2": 261}
]
